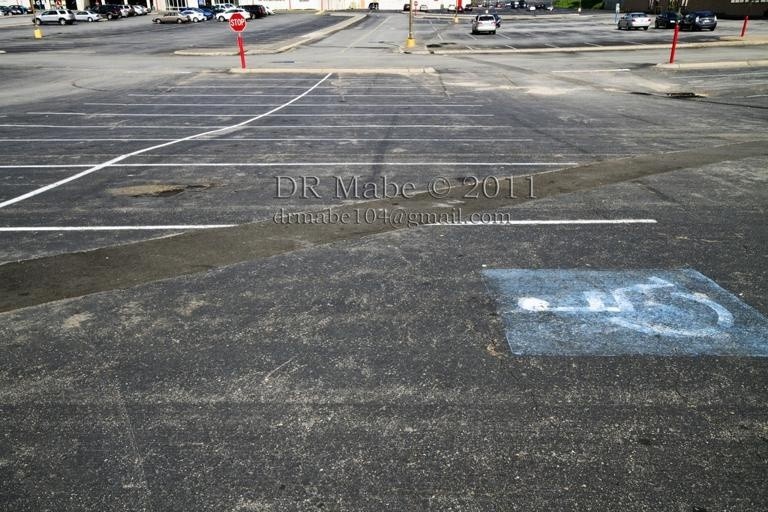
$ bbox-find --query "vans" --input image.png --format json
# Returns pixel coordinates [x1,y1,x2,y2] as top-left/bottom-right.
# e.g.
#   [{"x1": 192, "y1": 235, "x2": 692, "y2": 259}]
[
  {"x1": 471, "y1": 14, "x2": 496, "y2": 35},
  {"x1": 404, "y1": 4, "x2": 410, "y2": 12},
  {"x1": 236, "y1": 4, "x2": 266, "y2": 21},
  {"x1": 368, "y1": 2, "x2": 379, "y2": 10}
]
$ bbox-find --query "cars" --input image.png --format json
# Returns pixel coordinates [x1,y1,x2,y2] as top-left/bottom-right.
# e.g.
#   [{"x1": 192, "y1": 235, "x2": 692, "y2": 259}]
[
  {"x1": 655, "y1": 9, "x2": 691, "y2": 31},
  {"x1": 0, "y1": 5, "x2": 23, "y2": 16},
  {"x1": 152, "y1": 11, "x2": 189, "y2": 25},
  {"x1": 181, "y1": 10, "x2": 205, "y2": 23},
  {"x1": 446, "y1": 0, "x2": 555, "y2": 15},
  {"x1": 616, "y1": 11, "x2": 651, "y2": 32},
  {"x1": 117, "y1": 3, "x2": 151, "y2": 17},
  {"x1": 216, "y1": 8, "x2": 252, "y2": 22},
  {"x1": 8, "y1": 5, "x2": 33, "y2": 15},
  {"x1": 201, "y1": 2, "x2": 236, "y2": 20},
  {"x1": 263, "y1": 5, "x2": 272, "y2": 16},
  {"x1": 420, "y1": 4, "x2": 428, "y2": 11},
  {"x1": 72, "y1": 10, "x2": 102, "y2": 23},
  {"x1": 489, "y1": 13, "x2": 502, "y2": 28}
]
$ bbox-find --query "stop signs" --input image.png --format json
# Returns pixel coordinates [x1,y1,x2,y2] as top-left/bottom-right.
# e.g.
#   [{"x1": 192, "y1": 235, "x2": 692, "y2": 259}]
[
  {"x1": 414, "y1": 2, "x2": 418, "y2": 7},
  {"x1": 229, "y1": 13, "x2": 248, "y2": 33}
]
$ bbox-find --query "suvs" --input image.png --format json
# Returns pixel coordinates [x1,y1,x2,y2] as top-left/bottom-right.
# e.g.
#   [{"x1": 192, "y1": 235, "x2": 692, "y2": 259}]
[
  {"x1": 685, "y1": 10, "x2": 718, "y2": 32},
  {"x1": 184, "y1": 7, "x2": 213, "y2": 22},
  {"x1": 89, "y1": 4, "x2": 122, "y2": 21},
  {"x1": 32, "y1": 8, "x2": 76, "y2": 26}
]
